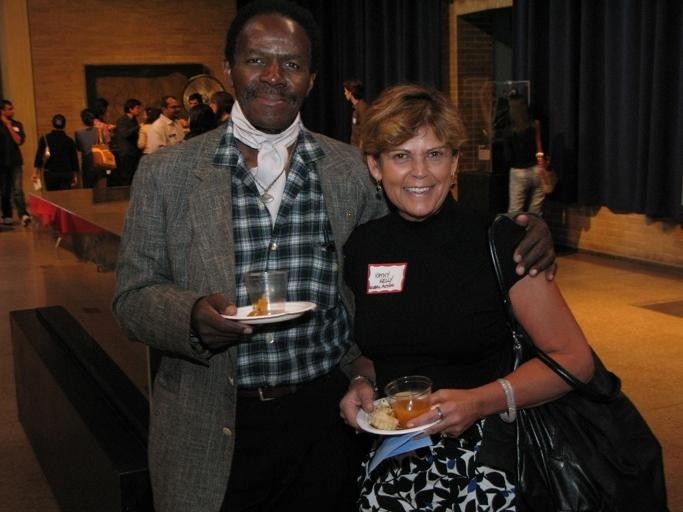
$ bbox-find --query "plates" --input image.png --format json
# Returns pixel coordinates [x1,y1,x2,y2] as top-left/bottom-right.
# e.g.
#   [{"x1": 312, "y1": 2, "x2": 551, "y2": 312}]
[
  {"x1": 222, "y1": 300, "x2": 317, "y2": 325},
  {"x1": 355, "y1": 396, "x2": 441, "y2": 436}
]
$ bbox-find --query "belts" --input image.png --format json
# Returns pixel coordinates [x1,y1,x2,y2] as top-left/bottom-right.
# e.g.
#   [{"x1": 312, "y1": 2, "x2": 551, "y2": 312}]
[{"x1": 237, "y1": 379, "x2": 325, "y2": 405}]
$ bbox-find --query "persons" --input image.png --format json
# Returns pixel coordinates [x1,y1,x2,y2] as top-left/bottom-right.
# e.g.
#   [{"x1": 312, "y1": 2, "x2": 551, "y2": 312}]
[
  {"x1": 339, "y1": 83, "x2": 596, "y2": 512},
  {"x1": 507, "y1": 94, "x2": 547, "y2": 217},
  {"x1": 0, "y1": 100, "x2": 32, "y2": 227},
  {"x1": 488, "y1": 97, "x2": 511, "y2": 214},
  {"x1": 33, "y1": 92, "x2": 235, "y2": 192},
  {"x1": 342, "y1": 79, "x2": 371, "y2": 146},
  {"x1": 112, "y1": 0, "x2": 557, "y2": 511}
]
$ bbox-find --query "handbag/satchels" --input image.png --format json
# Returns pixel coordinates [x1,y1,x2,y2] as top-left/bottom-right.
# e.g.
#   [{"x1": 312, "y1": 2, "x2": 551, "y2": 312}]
[
  {"x1": 89, "y1": 144, "x2": 117, "y2": 172},
  {"x1": 42, "y1": 146, "x2": 50, "y2": 164},
  {"x1": 536, "y1": 152, "x2": 558, "y2": 194},
  {"x1": 513, "y1": 337, "x2": 667, "y2": 512}
]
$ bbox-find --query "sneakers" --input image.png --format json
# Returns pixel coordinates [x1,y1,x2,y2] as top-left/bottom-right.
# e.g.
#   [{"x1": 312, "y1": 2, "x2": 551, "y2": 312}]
[{"x1": 4, "y1": 215, "x2": 31, "y2": 228}]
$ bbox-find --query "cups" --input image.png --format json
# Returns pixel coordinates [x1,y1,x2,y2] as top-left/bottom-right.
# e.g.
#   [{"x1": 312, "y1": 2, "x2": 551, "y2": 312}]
[
  {"x1": 385, "y1": 375, "x2": 433, "y2": 426},
  {"x1": 244, "y1": 271, "x2": 289, "y2": 315}
]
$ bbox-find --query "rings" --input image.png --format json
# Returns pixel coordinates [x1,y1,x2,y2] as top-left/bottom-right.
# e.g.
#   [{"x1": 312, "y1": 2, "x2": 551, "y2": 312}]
[{"x1": 436, "y1": 407, "x2": 443, "y2": 420}]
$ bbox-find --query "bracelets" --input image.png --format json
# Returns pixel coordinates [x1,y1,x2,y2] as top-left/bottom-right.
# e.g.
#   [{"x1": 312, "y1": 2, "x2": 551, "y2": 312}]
[
  {"x1": 496, "y1": 379, "x2": 517, "y2": 423},
  {"x1": 350, "y1": 375, "x2": 365, "y2": 381},
  {"x1": 536, "y1": 152, "x2": 545, "y2": 158}
]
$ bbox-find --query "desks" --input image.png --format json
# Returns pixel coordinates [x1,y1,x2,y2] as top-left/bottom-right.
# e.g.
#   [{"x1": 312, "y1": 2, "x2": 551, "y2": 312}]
[{"x1": 28, "y1": 185, "x2": 131, "y2": 248}]
